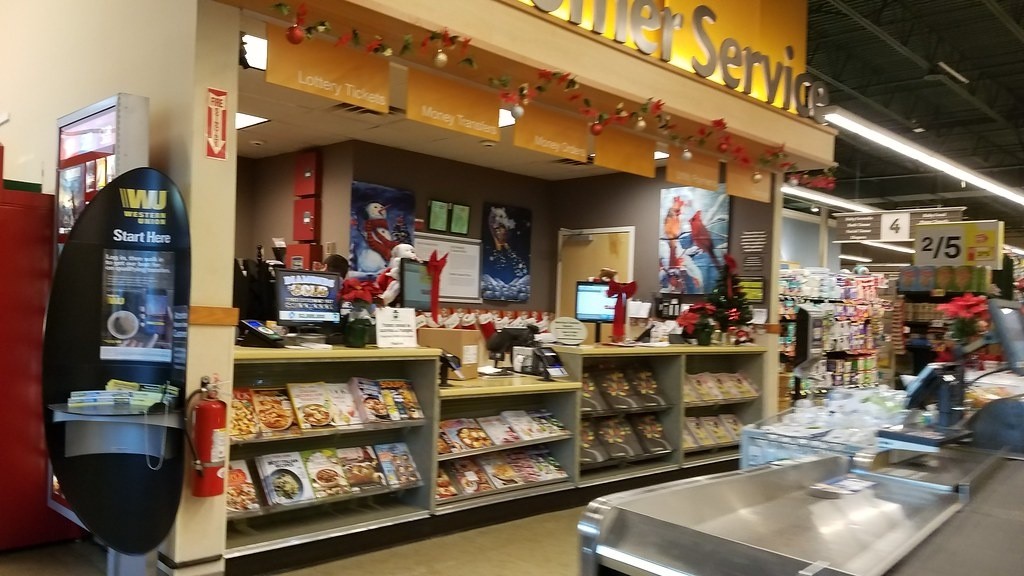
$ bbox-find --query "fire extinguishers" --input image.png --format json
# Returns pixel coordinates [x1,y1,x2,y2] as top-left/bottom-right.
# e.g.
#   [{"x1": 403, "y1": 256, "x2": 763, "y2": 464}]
[{"x1": 183, "y1": 375, "x2": 228, "y2": 497}]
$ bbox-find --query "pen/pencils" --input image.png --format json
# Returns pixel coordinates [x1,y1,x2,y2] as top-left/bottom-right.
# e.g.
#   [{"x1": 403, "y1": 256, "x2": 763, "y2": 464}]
[{"x1": 161, "y1": 380, "x2": 170, "y2": 402}]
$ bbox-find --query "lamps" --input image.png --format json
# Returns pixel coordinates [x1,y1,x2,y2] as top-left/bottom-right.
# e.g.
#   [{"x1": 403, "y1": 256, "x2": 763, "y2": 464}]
[{"x1": 780, "y1": 106, "x2": 1024, "y2": 267}]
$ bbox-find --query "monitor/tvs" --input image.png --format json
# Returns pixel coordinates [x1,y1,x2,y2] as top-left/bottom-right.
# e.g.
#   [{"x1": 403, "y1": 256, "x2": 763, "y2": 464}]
[
  {"x1": 989, "y1": 298, "x2": 1024, "y2": 377},
  {"x1": 486, "y1": 326, "x2": 533, "y2": 352},
  {"x1": 276, "y1": 268, "x2": 341, "y2": 335},
  {"x1": 576, "y1": 281, "x2": 626, "y2": 324}
]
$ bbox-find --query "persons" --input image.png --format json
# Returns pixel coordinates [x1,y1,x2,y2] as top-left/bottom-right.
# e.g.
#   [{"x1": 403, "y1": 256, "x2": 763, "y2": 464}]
[
  {"x1": 323, "y1": 255, "x2": 349, "y2": 289},
  {"x1": 901, "y1": 324, "x2": 948, "y2": 376}
]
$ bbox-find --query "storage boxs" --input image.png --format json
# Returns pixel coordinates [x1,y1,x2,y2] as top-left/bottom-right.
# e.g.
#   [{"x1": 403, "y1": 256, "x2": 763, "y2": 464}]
[
  {"x1": 899, "y1": 266, "x2": 992, "y2": 292},
  {"x1": 417, "y1": 327, "x2": 481, "y2": 380}
]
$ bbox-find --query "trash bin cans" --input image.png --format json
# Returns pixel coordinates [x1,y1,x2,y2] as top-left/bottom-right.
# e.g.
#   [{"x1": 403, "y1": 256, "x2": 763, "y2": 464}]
[{"x1": 904, "y1": 339, "x2": 935, "y2": 375}]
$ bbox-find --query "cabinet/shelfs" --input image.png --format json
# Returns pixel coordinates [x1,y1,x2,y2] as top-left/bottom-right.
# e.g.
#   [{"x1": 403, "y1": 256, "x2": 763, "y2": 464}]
[
  {"x1": 895, "y1": 290, "x2": 1016, "y2": 391},
  {"x1": 225, "y1": 332, "x2": 779, "y2": 576},
  {"x1": 416, "y1": 230, "x2": 484, "y2": 304}
]
